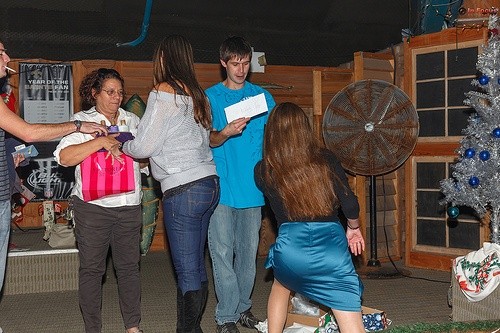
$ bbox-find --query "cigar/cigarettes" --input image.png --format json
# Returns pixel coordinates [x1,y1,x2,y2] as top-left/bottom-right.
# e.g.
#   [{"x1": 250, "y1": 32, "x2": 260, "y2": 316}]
[{"x1": 4, "y1": 66, "x2": 17, "y2": 74}]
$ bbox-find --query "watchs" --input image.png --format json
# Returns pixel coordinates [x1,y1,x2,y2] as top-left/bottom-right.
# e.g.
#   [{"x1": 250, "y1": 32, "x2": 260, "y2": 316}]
[{"x1": 74, "y1": 120, "x2": 81, "y2": 133}]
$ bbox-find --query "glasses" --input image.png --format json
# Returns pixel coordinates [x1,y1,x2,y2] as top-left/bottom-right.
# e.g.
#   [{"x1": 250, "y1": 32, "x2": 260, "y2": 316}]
[{"x1": 97, "y1": 88, "x2": 127, "y2": 97}]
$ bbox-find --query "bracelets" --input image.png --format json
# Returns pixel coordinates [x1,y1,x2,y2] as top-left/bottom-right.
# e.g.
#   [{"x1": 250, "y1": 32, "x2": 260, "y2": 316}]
[
  {"x1": 347, "y1": 224, "x2": 360, "y2": 229},
  {"x1": 118, "y1": 141, "x2": 124, "y2": 152}
]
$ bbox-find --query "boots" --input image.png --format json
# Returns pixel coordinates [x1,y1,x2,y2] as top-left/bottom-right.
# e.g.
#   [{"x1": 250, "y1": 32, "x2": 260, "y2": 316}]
[
  {"x1": 175, "y1": 287, "x2": 204, "y2": 333},
  {"x1": 192, "y1": 281, "x2": 209, "y2": 333}
]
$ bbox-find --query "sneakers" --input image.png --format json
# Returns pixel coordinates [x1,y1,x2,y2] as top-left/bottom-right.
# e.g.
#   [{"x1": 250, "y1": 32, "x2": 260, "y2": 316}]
[
  {"x1": 216, "y1": 321, "x2": 241, "y2": 333},
  {"x1": 238, "y1": 310, "x2": 262, "y2": 328}
]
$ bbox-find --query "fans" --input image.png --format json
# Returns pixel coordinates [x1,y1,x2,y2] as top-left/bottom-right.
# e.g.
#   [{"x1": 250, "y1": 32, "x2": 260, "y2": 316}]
[{"x1": 322, "y1": 79, "x2": 421, "y2": 279}]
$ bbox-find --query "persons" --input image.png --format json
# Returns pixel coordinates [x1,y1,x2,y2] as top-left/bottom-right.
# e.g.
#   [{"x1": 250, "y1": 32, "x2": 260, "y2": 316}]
[
  {"x1": 204, "y1": 38, "x2": 278, "y2": 333},
  {"x1": 53, "y1": 66, "x2": 150, "y2": 333},
  {"x1": 0, "y1": 40, "x2": 107, "y2": 333},
  {"x1": 104, "y1": 35, "x2": 220, "y2": 333},
  {"x1": 253, "y1": 102, "x2": 366, "y2": 333}
]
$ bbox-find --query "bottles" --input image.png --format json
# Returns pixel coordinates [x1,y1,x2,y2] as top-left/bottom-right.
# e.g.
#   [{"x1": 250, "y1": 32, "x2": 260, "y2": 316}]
[
  {"x1": 99, "y1": 119, "x2": 107, "y2": 132},
  {"x1": 110, "y1": 122, "x2": 119, "y2": 131},
  {"x1": 118, "y1": 119, "x2": 129, "y2": 132}
]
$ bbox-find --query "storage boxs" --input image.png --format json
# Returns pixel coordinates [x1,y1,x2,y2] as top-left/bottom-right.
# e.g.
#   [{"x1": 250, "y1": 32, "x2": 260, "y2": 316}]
[
  {"x1": 361, "y1": 305, "x2": 388, "y2": 333},
  {"x1": 284, "y1": 296, "x2": 340, "y2": 333}
]
